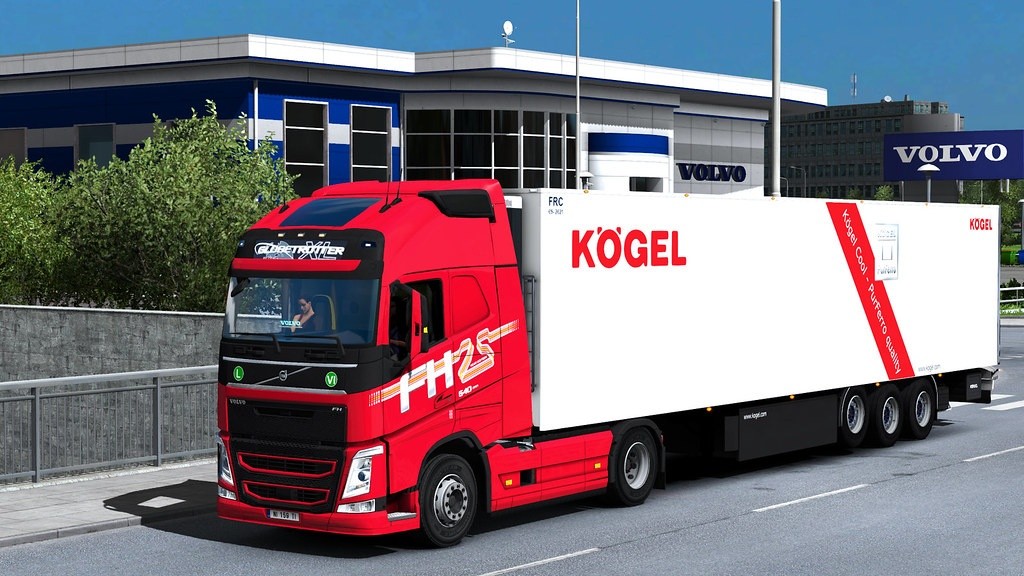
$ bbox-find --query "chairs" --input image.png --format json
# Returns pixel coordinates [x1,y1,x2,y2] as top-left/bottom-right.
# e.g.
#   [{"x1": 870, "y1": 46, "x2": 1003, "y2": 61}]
[{"x1": 311, "y1": 294, "x2": 337, "y2": 331}]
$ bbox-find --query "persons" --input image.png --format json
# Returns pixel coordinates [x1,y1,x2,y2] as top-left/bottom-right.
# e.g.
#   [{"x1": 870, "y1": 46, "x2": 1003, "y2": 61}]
[{"x1": 291, "y1": 295, "x2": 315, "y2": 333}]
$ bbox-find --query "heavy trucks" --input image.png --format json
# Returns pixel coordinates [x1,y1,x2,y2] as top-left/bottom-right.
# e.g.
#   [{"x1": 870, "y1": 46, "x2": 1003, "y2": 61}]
[{"x1": 217, "y1": 178, "x2": 1001, "y2": 551}]
